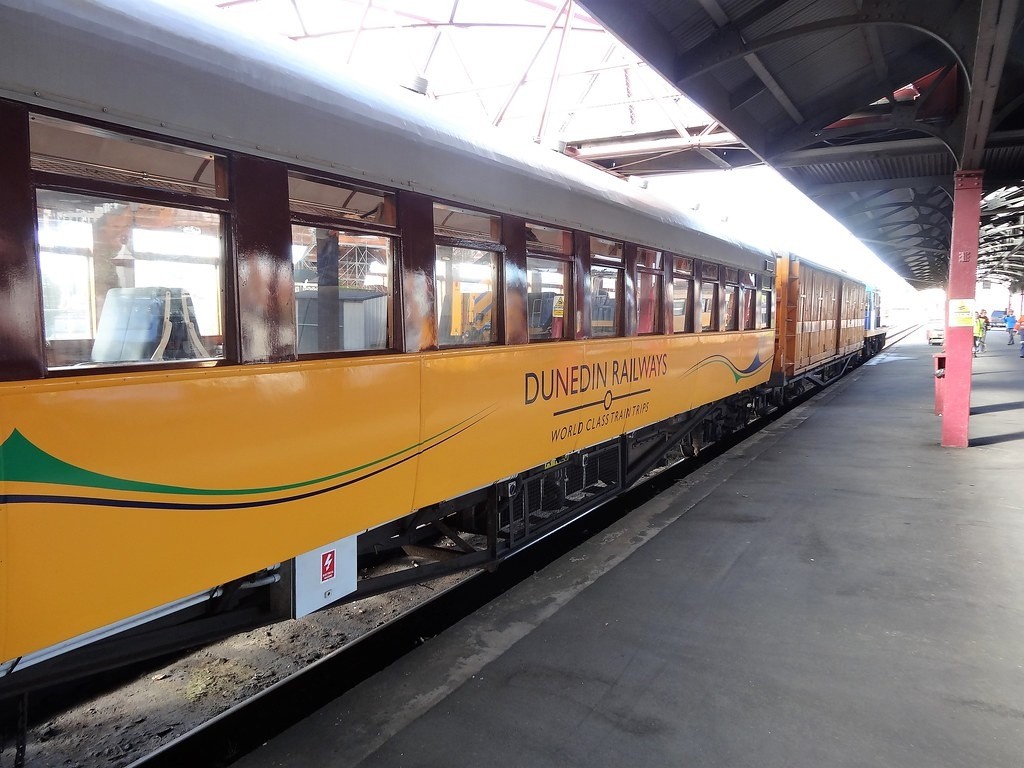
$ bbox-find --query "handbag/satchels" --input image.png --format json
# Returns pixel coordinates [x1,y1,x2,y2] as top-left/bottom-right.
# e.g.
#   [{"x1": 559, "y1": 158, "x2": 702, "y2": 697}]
[{"x1": 168, "y1": 309, "x2": 190, "y2": 359}]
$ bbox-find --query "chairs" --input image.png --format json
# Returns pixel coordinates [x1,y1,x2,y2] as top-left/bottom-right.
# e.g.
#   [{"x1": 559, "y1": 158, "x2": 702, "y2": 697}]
[
  {"x1": 523, "y1": 289, "x2": 618, "y2": 337},
  {"x1": 166, "y1": 286, "x2": 212, "y2": 361},
  {"x1": 74, "y1": 286, "x2": 176, "y2": 362}
]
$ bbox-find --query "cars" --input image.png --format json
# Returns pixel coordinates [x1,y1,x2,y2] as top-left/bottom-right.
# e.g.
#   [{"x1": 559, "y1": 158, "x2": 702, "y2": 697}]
[{"x1": 990, "y1": 310, "x2": 1007, "y2": 326}]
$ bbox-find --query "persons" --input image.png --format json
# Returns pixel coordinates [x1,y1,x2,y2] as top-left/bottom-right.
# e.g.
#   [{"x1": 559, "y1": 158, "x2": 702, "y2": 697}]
[
  {"x1": 973, "y1": 309, "x2": 991, "y2": 357},
  {"x1": 1003, "y1": 309, "x2": 1016, "y2": 344},
  {"x1": 1014, "y1": 315, "x2": 1024, "y2": 358}
]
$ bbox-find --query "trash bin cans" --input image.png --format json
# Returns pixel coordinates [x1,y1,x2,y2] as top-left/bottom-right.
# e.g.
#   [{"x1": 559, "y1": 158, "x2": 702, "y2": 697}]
[{"x1": 932, "y1": 350, "x2": 947, "y2": 417}]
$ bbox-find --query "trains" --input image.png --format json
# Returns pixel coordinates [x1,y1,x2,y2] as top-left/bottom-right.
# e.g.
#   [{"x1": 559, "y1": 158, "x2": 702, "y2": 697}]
[{"x1": 0, "y1": 1, "x2": 888, "y2": 737}]
